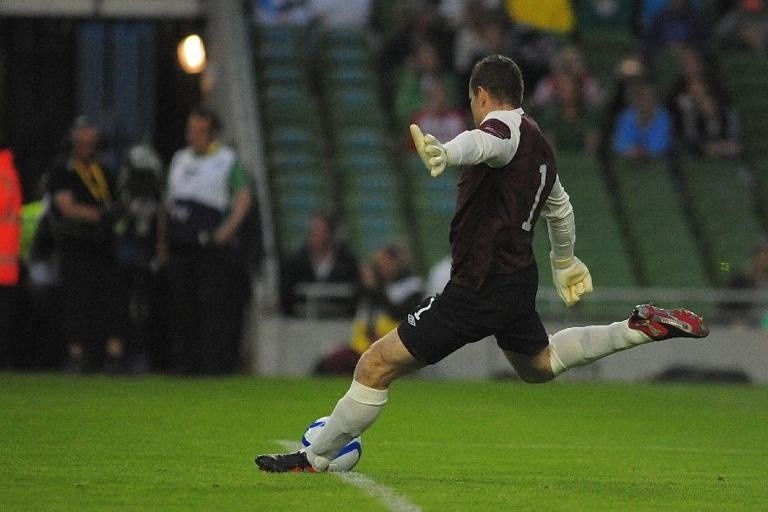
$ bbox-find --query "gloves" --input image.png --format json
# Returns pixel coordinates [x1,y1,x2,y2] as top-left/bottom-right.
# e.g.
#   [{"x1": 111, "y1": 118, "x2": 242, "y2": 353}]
[
  {"x1": 549, "y1": 250, "x2": 594, "y2": 308},
  {"x1": 409, "y1": 122, "x2": 448, "y2": 179}
]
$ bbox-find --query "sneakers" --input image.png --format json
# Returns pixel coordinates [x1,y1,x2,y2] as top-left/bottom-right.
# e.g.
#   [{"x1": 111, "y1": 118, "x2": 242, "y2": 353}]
[
  {"x1": 254, "y1": 446, "x2": 330, "y2": 473},
  {"x1": 626, "y1": 303, "x2": 711, "y2": 342}
]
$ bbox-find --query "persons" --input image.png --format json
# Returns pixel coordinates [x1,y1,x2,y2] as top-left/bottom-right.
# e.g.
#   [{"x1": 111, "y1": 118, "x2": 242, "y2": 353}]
[
  {"x1": 278, "y1": 214, "x2": 453, "y2": 375},
  {"x1": 1, "y1": 106, "x2": 255, "y2": 380},
  {"x1": 256, "y1": 1, "x2": 766, "y2": 167},
  {"x1": 717, "y1": 248, "x2": 768, "y2": 328},
  {"x1": 254, "y1": 52, "x2": 711, "y2": 474}
]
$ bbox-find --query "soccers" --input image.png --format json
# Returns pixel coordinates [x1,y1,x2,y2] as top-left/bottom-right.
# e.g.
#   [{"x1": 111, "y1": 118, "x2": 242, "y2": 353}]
[{"x1": 301, "y1": 417, "x2": 362, "y2": 471}]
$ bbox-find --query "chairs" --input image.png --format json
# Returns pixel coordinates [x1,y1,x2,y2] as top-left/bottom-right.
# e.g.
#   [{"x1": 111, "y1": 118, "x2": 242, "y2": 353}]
[{"x1": 260, "y1": 22, "x2": 767, "y2": 323}]
